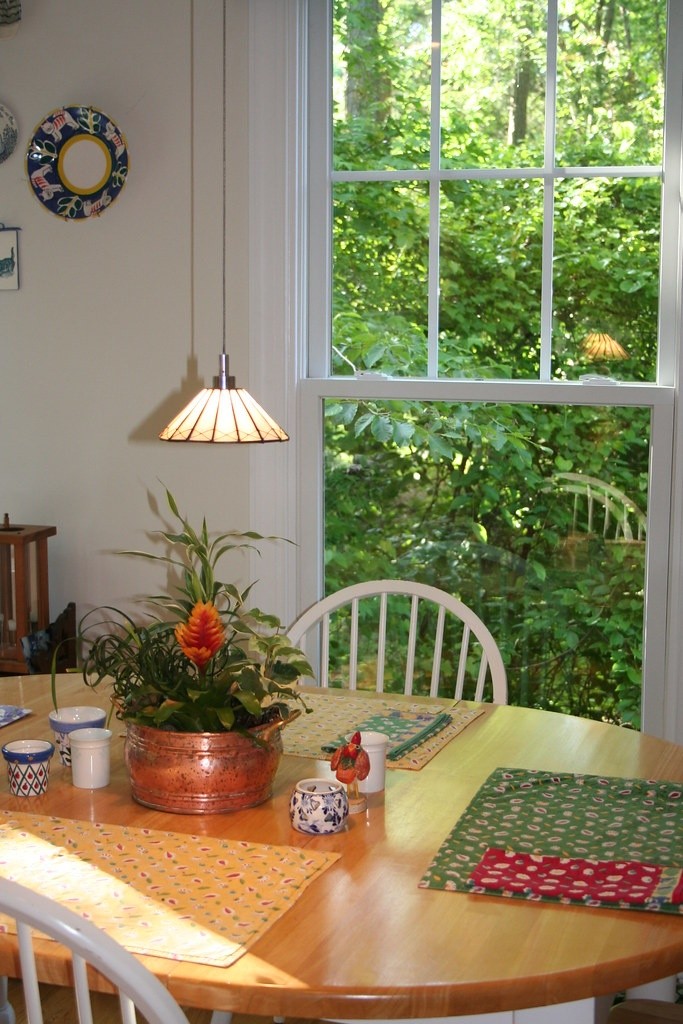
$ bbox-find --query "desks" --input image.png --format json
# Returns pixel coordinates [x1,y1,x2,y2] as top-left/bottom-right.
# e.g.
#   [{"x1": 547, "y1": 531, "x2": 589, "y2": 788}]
[{"x1": 0, "y1": 674, "x2": 683, "y2": 1024}]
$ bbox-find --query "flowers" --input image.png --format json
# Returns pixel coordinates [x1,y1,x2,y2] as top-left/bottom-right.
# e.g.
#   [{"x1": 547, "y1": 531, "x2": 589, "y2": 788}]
[
  {"x1": 330, "y1": 730, "x2": 370, "y2": 798},
  {"x1": 48, "y1": 474, "x2": 318, "y2": 726}
]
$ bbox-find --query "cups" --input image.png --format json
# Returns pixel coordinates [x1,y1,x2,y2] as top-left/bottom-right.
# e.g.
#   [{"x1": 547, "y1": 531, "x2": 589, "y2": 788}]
[
  {"x1": 289, "y1": 779, "x2": 349, "y2": 836},
  {"x1": 2, "y1": 739, "x2": 55, "y2": 797},
  {"x1": 49, "y1": 706, "x2": 107, "y2": 766},
  {"x1": 69, "y1": 728, "x2": 113, "y2": 789},
  {"x1": 344, "y1": 731, "x2": 390, "y2": 794}
]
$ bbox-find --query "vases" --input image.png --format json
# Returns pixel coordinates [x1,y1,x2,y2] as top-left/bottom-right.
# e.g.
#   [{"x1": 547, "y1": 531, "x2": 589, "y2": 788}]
[
  {"x1": 344, "y1": 791, "x2": 368, "y2": 815},
  {"x1": 110, "y1": 692, "x2": 301, "y2": 815}
]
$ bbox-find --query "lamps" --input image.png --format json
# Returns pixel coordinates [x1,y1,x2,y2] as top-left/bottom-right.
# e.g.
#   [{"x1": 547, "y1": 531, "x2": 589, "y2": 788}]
[
  {"x1": 579, "y1": 331, "x2": 632, "y2": 362},
  {"x1": 157, "y1": 0, "x2": 289, "y2": 443}
]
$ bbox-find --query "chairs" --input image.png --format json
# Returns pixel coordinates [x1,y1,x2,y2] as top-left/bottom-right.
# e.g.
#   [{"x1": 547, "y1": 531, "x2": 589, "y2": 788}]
[
  {"x1": 283, "y1": 579, "x2": 509, "y2": 706},
  {"x1": 0, "y1": 876, "x2": 189, "y2": 1024}
]
